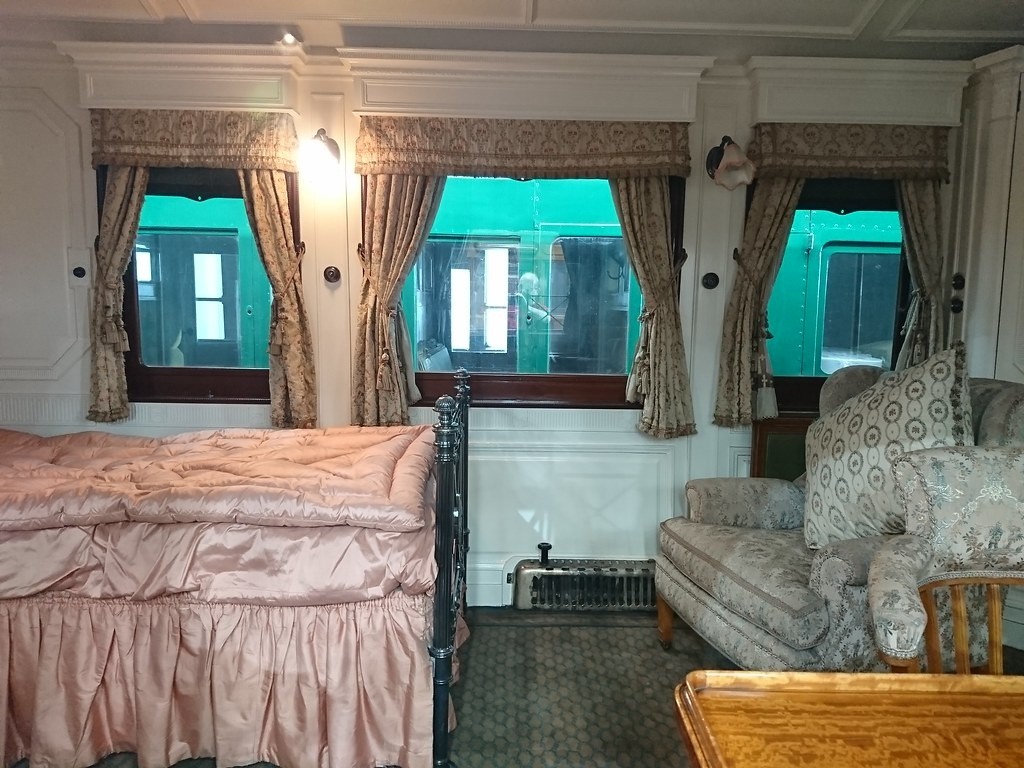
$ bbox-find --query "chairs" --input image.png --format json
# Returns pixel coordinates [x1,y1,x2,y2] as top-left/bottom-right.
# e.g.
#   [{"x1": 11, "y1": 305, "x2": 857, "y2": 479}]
[{"x1": 899, "y1": 566, "x2": 1024, "y2": 675}]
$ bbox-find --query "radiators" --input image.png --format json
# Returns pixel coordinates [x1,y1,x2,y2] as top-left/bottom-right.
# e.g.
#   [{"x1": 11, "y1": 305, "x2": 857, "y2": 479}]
[{"x1": 506, "y1": 540, "x2": 661, "y2": 614}]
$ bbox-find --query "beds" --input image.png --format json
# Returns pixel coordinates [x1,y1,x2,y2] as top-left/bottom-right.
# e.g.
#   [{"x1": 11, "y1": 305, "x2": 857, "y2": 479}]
[{"x1": 1, "y1": 365, "x2": 473, "y2": 768}]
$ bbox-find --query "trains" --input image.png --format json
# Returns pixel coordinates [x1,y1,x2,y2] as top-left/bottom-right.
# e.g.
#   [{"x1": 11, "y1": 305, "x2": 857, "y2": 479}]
[{"x1": 114, "y1": 175, "x2": 907, "y2": 379}]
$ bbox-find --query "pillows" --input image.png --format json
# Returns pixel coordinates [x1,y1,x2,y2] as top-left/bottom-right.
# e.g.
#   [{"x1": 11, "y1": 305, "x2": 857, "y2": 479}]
[{"x1": 800, "y1": 339, "x2": 975, "y2": 551}]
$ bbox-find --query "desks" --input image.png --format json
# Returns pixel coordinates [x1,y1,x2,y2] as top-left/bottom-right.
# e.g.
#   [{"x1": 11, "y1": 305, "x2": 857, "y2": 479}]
[{"x1": 672, "y1": 668, "x2": 1024, "y2": 768}]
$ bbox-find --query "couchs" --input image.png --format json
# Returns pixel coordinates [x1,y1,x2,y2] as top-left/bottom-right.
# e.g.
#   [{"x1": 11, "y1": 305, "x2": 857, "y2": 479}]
[{"x1": 652, "y1": 362, "x2": 1024, "y2": 671}]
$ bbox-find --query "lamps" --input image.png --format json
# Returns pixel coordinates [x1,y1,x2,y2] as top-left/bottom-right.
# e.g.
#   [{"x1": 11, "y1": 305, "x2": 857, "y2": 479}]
[{"x1": 706, "y1": 135, "x2": 757, "y2": 190}]
